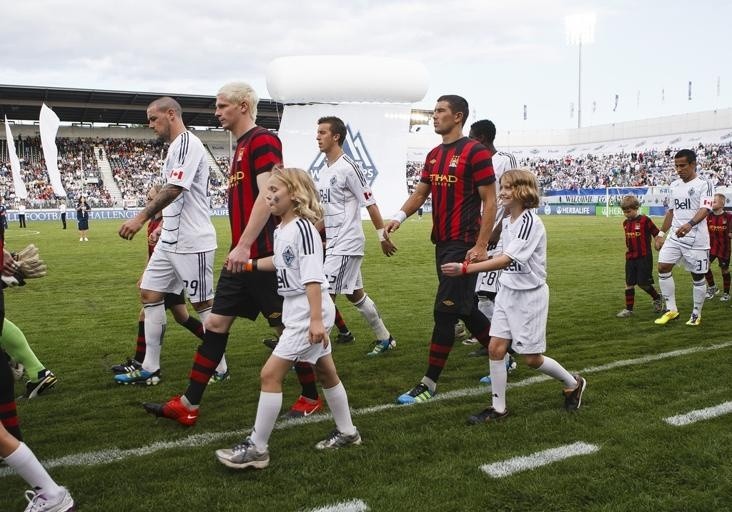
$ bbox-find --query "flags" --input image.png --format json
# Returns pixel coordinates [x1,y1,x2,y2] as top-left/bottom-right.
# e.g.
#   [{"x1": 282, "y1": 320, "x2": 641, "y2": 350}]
[
  {"x1": 277, "y1": 102, "x2": 410, "y2": 221},
  {"x1": 40, "y1": 103, "x2": 67, "y2": 198},
  {"x1": 4, "y1": 114, "x2": 26, "y2": 200}
]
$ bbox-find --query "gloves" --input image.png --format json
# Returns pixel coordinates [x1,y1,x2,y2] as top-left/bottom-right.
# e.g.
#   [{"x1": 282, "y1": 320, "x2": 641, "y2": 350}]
[{"x1": 1, "y1": 244, "x2": 47, "y2": 288}]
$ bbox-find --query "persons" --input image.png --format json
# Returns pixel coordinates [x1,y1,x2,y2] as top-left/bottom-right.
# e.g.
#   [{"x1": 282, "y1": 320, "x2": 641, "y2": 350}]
[
  {"x1": 27, "y1": 181, "x2": 56, "y2": 208},
  {"x1": 214, "y1": 169, "x2": 361, "y2": 471},
  {"x1": 20, "y1": 157, "x2": 49, "y2": 180},
  {"x1": 442, "y1": 170, "x2": 585, "y2": 427},
  {"x1": 110, "y1": 183, "x2": 205, "y2": 374},
  {"x1": 698, "y1": 193, "x2": 732, "y2": 302},
  {"x1": 59, "y1": 201, "x2": 67, "y2": 232},
  {"x1": 418, "y1": 205, "x2": 424, "y2": 220},
  {"x1": 18, "y1": 201, "x2": 27, "y2": 228},
  {"x1": 455, "y1": 120, "x2": 517, "y2": 344},
  {"x1": 317, "y1": 117, "x2": 397, "y2": 357},
  {"x1": 0, "y1": 248, "x2": 57, "y2": 401},
  {"x1": 0, "y1": 161, "x2": 20, "y2": 207},
  {"x1": 142, "y1": 82, "x2": 322, "y2": 429},
  {"x1": 615, "y1": 196, "x2": 663, "y2": 318},
  {"x1": 654, "y1": 150, "x2": 713, "y2": 325},
  {"x1": 0, "y1": 207, "x2": 23, "y2": 466},
  {"x1": 56, "y1": 137, "x2": 169, "y2": 208},
  {"x1": 17, "y1": 133, "x2": 42, "y2": 150},
  {"x1": 520, "y1": 147, "x2": 680, "y2": 194},
  {"x1": 75, "y1": 195, "x2": 91, "y2": 243},
  {"x1": 382, "y1": 95, "x2": 518, "y2": 406},
  {"x1": 0, "y1": 423, "x2": 76, "y2": 512},
  {"x1": 209, "y1": 141, "x2": 236, "y2": 208},
  {"x1": 0, "y1": 197, "x2": 8, "y2": 246},
  {"x1": 407, "y1": 161, "x2": 424, "y2": 194},
  {"x1": 113, "y1": 99, "x2": 229, "y2": 387},
  {"x1": 263, "y1": 219, "x2": 355, "y2": 350},
  {"x1": 692, "y1": 143, "x2": 732, "y2": 187}
]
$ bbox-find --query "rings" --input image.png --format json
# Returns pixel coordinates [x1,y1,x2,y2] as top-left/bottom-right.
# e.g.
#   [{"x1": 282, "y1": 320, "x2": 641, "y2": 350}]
[{"x1": 682, "y1": 232, "x2": 685, "y2": 235}]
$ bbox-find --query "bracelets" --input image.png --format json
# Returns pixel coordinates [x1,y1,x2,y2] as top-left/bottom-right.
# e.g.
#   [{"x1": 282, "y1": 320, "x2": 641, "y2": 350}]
[
  {"x1": 462, "y1": 260, "x2": 468, "y2": 275},
  {"x1": 246, "y1": 259, "x2": 252, "y2": 272},
  {"x1": 252, "y1": 258, "x2": 257, "y2": 273}
]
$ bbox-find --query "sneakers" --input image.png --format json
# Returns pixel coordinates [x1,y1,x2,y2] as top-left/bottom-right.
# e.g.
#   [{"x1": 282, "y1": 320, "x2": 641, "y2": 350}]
[
  {"x1": 469, "y1": 346, "x2": 489, "y2": 357},
  {"x1": 562, "y1": 375, "x2": 586, "y2": 413},
  {"x1": 653, "y1": 292, "x2": 664, "y2": 313},
  {"x1": 686, "y1": 314, "x2": 701, "y2": 326},
  {"x1": 705, "y1": 285, "x2": 720, "y2": 299},
  {"x1": 480, "y1": 354, "x2": 517, "y2": 384},
  {"x1": 0, "y1": 459, "x2": 9, "y2": 471},
  {"x1": 396, "y1": 383, "x2": 437, "y2": 405},
  {"x1": 616, "y1": 309, "x2": 632, "y2": 318},
  {"x1": 215, "y1": 437, "x2": 270, "y2": 469},
  {"x1": 367, "y1": 334, "x2": 396, "y2": 357},
  {"x1": 25, "y1": 486, "x2": 74, "y2": 512},
  {"x1": 333, "y1": 331, "x2": 355, "y2": 344},
  {"x1": 17, "y1": 369, "x2": 57, "y2": 402},
  {"x1": 207, "y1": 368, "x2": 229, "y2": 386},
  {"x1": 720, "y1": 293, "x2": 730, "y2": 302},
  {"x1": 462, "y1": 336, "x2": 480, "y2": 345},
  {"x1": 454, "y1": 322, "x2": 466, "y2": 338},
  {"x1": 111, "y1": 359, "x2": 140, "y2": 373},
  {"x1": 315, "y1": 426, "x2": 362, "y2": 452},
  {"x1": 280, "y1": 394, "x2": 323, "y2": 420},
  {"x1": 654, "y1": 310, "x2": 679, "y2": 325},
  {"x1": 113, "y1": 368, "x2": 161, "y2": 386},
  {"x1": 466, "y1": 407, "x2": 510, "y2": 426},
  {"x1": 141, "y1": 394, "x2": 198, "y2": 426},
  {"x1": 12, "y1": 363, "x2": 25, "y2": 382}
]
